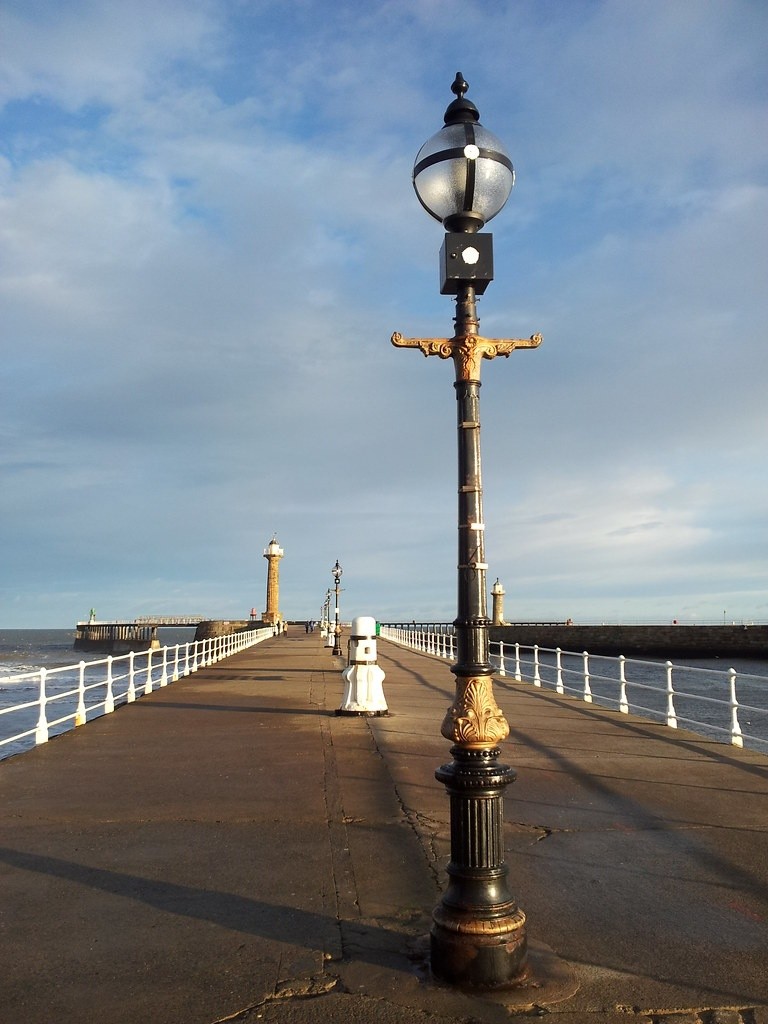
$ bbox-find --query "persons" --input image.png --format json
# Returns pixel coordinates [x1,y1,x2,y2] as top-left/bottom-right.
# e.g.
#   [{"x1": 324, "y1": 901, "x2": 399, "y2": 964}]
[
  {"x1": 276, "y1": 621, "x2": 281, "y2": 635},
  {"x1": 304, "y1": 621, "x2": 309, "y2": 633},
  {"x1": 283, "y1": 621, "x2": 288, "y2": 636},
  {"x1": 309, "y1": 619, "x2": 315, "y2": 632}
]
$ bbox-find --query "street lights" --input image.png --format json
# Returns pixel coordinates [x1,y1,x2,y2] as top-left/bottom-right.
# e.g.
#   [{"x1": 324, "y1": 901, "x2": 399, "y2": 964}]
[
  {"x1": 389, "y1": 70, "x2": 547, "y2": 998},
  {"x1": 328, "y1": 559, "x2": 347, "y2": 656},
  {"x1": 322, "y1": 589, "x2": 336, "y2": 648}
]
